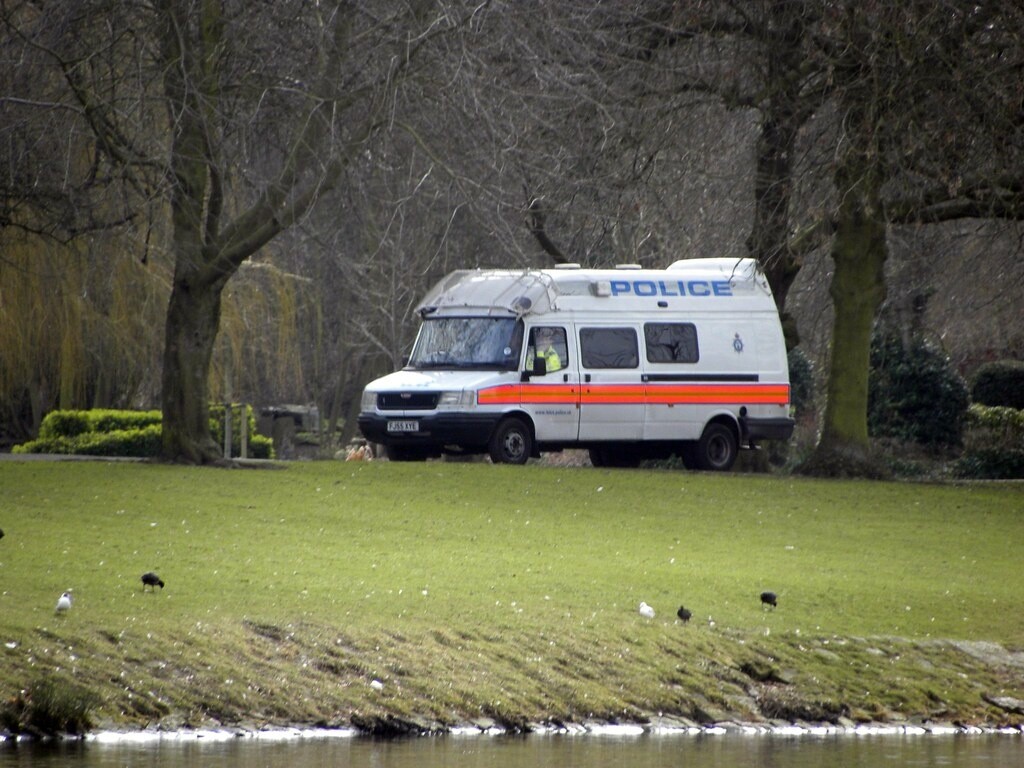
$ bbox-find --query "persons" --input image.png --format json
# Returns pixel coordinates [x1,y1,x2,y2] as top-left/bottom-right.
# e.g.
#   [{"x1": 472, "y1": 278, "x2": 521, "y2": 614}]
[{"x1": 525, "y1": 328, "x2": 561, "y2": 372}]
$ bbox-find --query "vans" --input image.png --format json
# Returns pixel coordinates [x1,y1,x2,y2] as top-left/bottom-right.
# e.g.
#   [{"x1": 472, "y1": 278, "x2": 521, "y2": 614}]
[{"x1": 357, "y1": 257, "x2": 795, "y2": 474}]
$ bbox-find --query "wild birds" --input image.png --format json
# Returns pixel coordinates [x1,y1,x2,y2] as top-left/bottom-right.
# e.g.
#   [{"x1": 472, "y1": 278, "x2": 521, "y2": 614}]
[
  {"x1": 54, "y1": 588, "x2": 74, "y2": 615},
  {"x1": 142, "y1": 571, "x2": 166, "y2": 592},
  {"x1": 639, "y1": 602, "x2": 656, "y2": 619},
  {"x1": 760, "y1": 591, "x2": 777, "y2": 609},
  {"x1": 677, "y1": 604, "x2": 692, "y2": 622}
]
